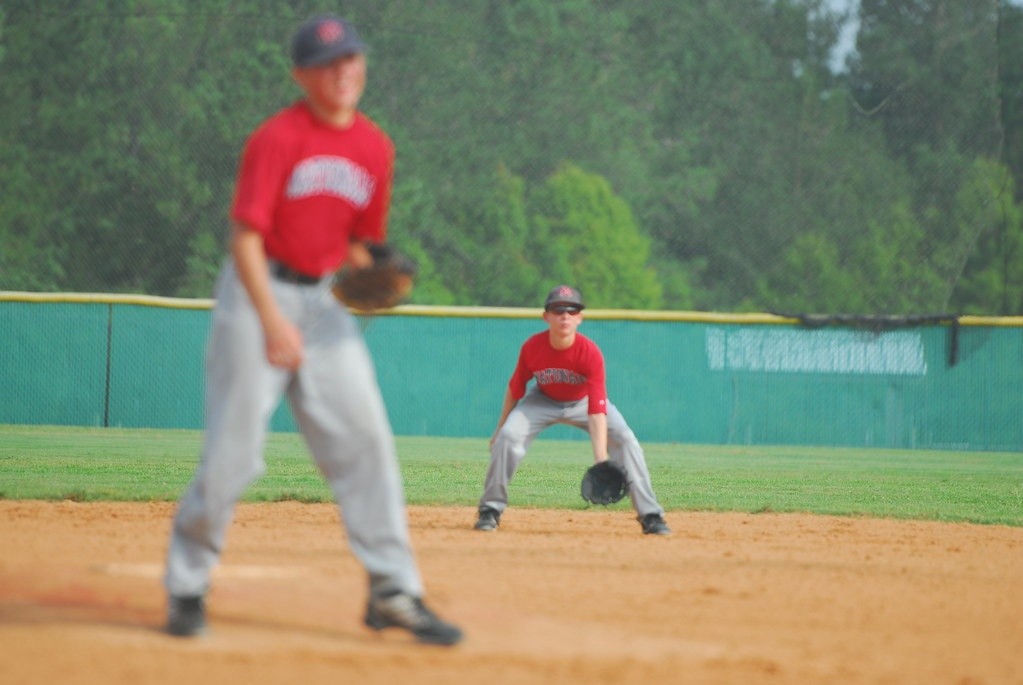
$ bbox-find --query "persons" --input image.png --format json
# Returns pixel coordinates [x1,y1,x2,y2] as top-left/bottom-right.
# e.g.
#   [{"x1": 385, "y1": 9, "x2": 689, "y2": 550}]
[
  {"x1": 476, "y1": 284, "x2": 671, "y2": 536},
  {"x1": 163, "y1": 15, "x2": 460, "y2": 642}
]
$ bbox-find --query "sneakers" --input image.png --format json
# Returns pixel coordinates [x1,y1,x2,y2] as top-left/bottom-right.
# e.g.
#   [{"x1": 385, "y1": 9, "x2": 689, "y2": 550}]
[
  {"x1": 472, "y1": 505, "x2": 500, "y2": 532},
  {"x1": 170, "y1": 591, "x2": 208, "y2": 639},
  {"x1": 636, "y1": 515, "x2": 671, "y2": 535},
  {"x1": 363, "y1": 589, "x2": 463, "y2": 648}
]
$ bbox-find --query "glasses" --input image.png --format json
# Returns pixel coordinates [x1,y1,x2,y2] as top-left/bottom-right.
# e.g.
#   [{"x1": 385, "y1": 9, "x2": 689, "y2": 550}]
[{"x1": 549, "y1": 306, "x2": 581, "y2": 315}]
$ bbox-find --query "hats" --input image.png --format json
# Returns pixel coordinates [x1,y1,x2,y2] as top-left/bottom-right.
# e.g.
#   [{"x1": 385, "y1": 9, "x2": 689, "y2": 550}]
[
  {"x1": 544, "y1": 284, "x2": 586, "y2": 309},
  {"x1": 289, "y1": 14, "x2": 364, "y2": 68}
]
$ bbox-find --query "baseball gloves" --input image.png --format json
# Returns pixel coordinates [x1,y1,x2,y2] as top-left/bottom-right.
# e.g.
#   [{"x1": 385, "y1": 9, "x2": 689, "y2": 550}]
[
  {"x1": 579, "y1": 459, "x2": 633, "y2": 508},
  {"x1": 329, "y1": 240, "x2": 418, "y2": 312}
]
